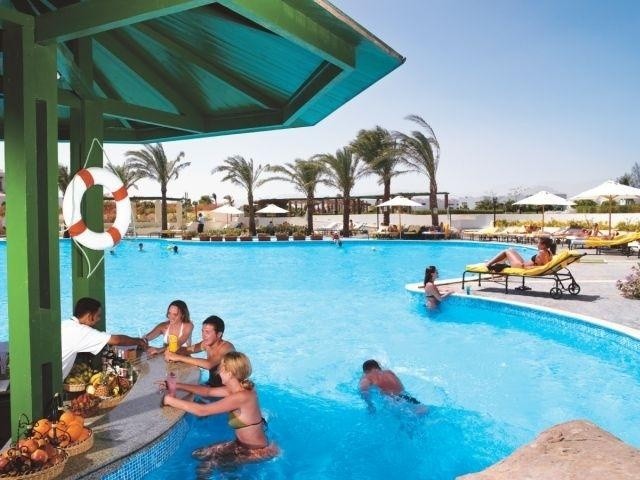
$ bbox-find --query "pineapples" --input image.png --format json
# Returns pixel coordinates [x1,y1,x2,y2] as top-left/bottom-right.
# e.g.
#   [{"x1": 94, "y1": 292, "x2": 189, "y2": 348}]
[{"x1": 95, "y1": 374, "x2": 110, "y2": 397}]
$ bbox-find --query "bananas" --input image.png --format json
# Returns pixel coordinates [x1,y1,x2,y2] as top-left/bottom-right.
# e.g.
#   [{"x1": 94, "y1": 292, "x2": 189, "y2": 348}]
[{"x1": 90, "y1": 372, "x2": 100, "y2": 386}]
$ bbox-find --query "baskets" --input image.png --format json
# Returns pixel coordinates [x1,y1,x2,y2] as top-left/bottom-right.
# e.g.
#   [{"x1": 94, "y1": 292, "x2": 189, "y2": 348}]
[
  {"x1": 0, "y1": 413, "x2": 70, "y2": 480},
  {"x1": 97, "y1": 389, "x2": 131, "y2": 410},
  {"x1": 62, "y1": 382, "x2": 86, "y2": 393},
  {"x1": 51, "y1": 393, "x2": 95, "y2": 458}
]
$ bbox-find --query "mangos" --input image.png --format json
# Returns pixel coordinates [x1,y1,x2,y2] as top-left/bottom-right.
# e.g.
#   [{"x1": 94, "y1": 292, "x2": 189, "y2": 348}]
[
  {"x1": 66, "y1": 424, "x2": 82, "y2": 441},
  {"x1": 33, "y1": 419, "x2": 50, "y2": 437},
  {"x1": 59, "y1": 411, "x2": 73, "y2": 425},
  {"x1": 76, "y1": 428, "x2": 88, "y2": 442},
  {"x1": 72, "y1": 416, "x2": 84, "y2": 425},
  {"x1": 49, "y1": 426, "x2": 69, "y2": 445}
]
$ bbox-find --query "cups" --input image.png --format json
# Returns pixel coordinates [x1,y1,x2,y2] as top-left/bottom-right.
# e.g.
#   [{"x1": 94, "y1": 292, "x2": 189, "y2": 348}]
[
  {"x1": 167, "y1": 372, "x2": 178, "y2": 397},
  {"x1": 466, "y1": 285, "x2": 470, "y2": 295},
  {"x1": 138, "y1": 327, "x2": 145, "y2": 339},
  {"x1": 473, "y1": 288, "x2": 476, "y2": 294},
  {"x1": 169, "y1": 336, "x2": 178, "y2": 353}
]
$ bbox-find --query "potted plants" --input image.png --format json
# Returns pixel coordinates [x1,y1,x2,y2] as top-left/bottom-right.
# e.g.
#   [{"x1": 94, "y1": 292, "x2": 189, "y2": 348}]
[
  {"x1": 181, "y1": 231, "x2": 195, "y2": 240},
  {"x1": 258, "y1": 231, "x2": 270, "y2": 242},
  {"x1": 311, "y1": 231, "x2": 323, "y2": 240},
  {"x1": 277, "y1": 231, "x2": 288, "y2": 242},
  {"x1": 239, "y1": 228, "x2": 252, "y2": 241},
  {"x1": 200, "y1": 230, "x2": 212, "y2": 242},
  {"x1": 211, "y1": 228, "x2": 223, "y2": 241},
  {"x1": 224, "y1": 229, "x2": 237, "y2": 241},
  {"x1": 292, "y1": 230, "x2": 304, "y2": 240}
]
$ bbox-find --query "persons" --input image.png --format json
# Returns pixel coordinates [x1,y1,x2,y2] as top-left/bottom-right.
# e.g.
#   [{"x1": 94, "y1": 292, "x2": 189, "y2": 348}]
[
  {"x1": 583, "y1": 222, "x2": 619, "y2": 241},
  {"x1": 137, "y1": 243, "x2": 144, "y2": 251},
  {"x1": 163, "y1": 315, "x2": 236, "y2": 388},
  {"x1": 349, "y1": 219, "x2": 354, "y2": 230},
  {"x1": 486, "y1": 237, "x2": 557, "y2": 269},
  {"x1": 424, "y1": 265, "x2": 455, "y2": 308},
  {"x1": 236, "y1": 222, "x2": 243, "y2": 229},
  {"x1": 172, "y1": 246, "x2": 178, "y2": 254},
  {"x1": 196, "y1": 212, "x2": 205, "y2": 233},
  {"x1": 267, "y1": 220, "x2": 274, "y2": 227},
  {"x1": 332, "y1": 232, "x2": 339, "y2": 245},
  {"x1": 61, "y1": 297, "x2": 148, "y2": 384},
  {"x1": 142, "y1": 299, "x2": 194, "y2": 355},
  {"x1": 155, "y1": 350, "x2": 280, "y2": 480},
  {"x1": 388, "y1": 225, "x2": 398, "y2": 237},
  {"x1": 357, "y1": 359, "x2": 422, "y2": 415}
]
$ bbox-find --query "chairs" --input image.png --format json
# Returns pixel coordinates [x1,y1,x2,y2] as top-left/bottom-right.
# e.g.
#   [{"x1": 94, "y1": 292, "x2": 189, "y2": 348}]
[
  {"x1": 465, "y1": 228, "x2": 625, "y2": 254},
  {"x1": 585, "y1": 234, "x2": 639, "y2": 254},
  {"x1": 461, "y1": 250, "x2": 585, "y2": 299}
]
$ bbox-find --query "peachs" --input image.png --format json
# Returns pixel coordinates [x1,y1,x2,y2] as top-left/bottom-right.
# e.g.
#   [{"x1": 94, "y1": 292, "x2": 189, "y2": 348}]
[{"x1": 0, "y1": 438, "x2": 61, "y2": 472}]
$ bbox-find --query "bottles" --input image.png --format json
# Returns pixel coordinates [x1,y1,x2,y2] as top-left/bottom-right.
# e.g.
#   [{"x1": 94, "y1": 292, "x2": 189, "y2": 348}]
[
  {"x1": 100, "y1": 344, "x2": 134, "y2": 385},
  {"x1": 6, "y1": 353, "x2": 10, "y2": 379}
]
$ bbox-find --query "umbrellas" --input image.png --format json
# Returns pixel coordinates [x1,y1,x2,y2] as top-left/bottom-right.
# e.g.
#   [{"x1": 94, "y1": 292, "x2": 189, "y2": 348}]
[
  {"x1": 210, "y1": 203, "x2": 244, "y2": 229},
  {"x1": 512, "y1": 189, "x2": 578, "y2": 233},
  {"x1": 255, "y1": 203, "x2": 290, "y2": 224},
  {"x1": 374, "y1": 195, "x2": 423, "y2": 239},
  {"x1": 568, "y1": 180, "x2": 640, "y2": 236}
]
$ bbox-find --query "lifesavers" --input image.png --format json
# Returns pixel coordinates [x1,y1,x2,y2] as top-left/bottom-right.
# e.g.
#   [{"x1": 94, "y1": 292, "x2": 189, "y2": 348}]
[{"x1": 62, "y1": 166, "x2": 133, "y2": 252}]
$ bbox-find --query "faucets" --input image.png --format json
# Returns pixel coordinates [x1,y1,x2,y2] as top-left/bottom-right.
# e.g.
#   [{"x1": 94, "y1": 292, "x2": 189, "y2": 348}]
[{"x1": 104, "y1": 362, "x2": 118, "y2": 374}]
